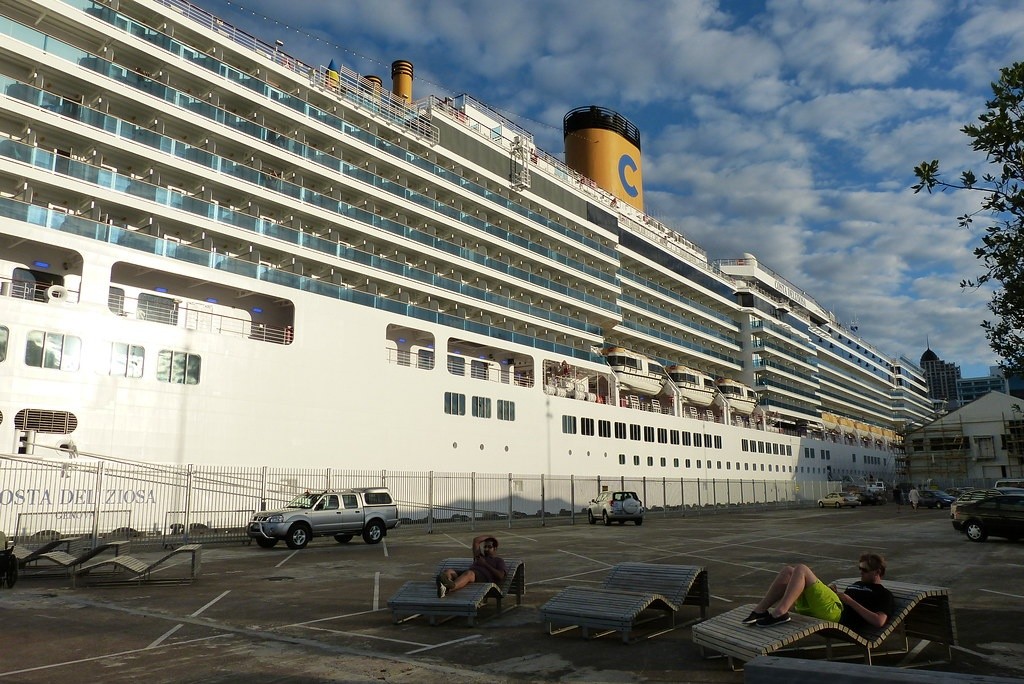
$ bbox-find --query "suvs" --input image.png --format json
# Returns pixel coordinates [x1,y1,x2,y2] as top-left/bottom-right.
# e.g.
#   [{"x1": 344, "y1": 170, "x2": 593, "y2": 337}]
[{"x1": 585, "y1": 490, "x2": 644, "y2": 527}]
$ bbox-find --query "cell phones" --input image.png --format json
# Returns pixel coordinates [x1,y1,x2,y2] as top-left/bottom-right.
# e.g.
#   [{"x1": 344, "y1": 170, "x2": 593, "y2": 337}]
[{"x1": 476, "y1": 555, "x2": 480, "y2": 560}]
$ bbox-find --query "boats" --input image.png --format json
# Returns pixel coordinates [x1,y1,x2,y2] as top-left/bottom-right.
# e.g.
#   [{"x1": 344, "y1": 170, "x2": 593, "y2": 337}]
[
  {"x1": 668, "y1": 364, "x2": 717, "y2": 408},
  {"x1": 600, "y1": 346, "x2": 668, "y2": 398},
  {"x1": 867, "y1": 425, "x2": 883, "y2": 440},
  {"x1": 851, "y1": 421, "x2": 870, "y2": 438},
  {"x1": 821, "y1": 411, "x2": 837, "y2": 430},
  {"x1": 881, "y1": 429, "x2": 894, "y2": 443},
  {"x1": 835, "y1": 417, "x2": 854, "y2": 435},
  {"x1": 715, "y1": 377, "x2": 758, "y2": 416}
]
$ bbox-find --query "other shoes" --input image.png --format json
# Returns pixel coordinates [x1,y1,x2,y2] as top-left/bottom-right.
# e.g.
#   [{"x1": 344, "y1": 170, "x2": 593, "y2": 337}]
[
  {"x1": 436, "y1": 580, "x2": 447, "y2": 598},
  {"x1": 438, "y1": 572, "x2": 456, "y2": 589}
]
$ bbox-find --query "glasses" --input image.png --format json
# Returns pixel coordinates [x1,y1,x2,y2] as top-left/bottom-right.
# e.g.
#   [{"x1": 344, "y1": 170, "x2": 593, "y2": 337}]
[
  {"x1": 859, "y1": 566, "x2": 873, "y2": 573},
  {"x1": 483, "y1": 544, "x2": 494, "y2": 548}
]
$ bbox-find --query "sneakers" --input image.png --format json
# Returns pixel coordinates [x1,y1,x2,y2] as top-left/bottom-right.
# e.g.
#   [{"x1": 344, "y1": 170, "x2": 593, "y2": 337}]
[
  {"x1": 742, "y1": 609, "x2": 771, "y2": 624},
  {"x1": 756, "y1": 611, "x2": 792, "y2": 627}
]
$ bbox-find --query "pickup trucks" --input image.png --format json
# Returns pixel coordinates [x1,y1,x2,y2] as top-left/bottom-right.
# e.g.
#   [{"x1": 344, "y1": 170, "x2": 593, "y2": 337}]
[{"x1": 244, "y1": 486, "x2": 403, "y2": 551}]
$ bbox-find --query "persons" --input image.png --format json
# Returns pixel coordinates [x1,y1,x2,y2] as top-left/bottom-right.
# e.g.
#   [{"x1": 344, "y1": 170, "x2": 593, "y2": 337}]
[
  {"x1": 910, "y1": 487, "x2": 921, "y2": 512},
  {"x1": 892, "y1": 485, "x2": 903, "y2": 513},
  {"x1": 869, "y1": 472, "x2": 873, "y2": 480},
  {"x1": 742, "y1": 552, "x2": 894, "y2": 635},
  {"x1": 436, "y1": 535, "x2": 506, "y2": 599}
]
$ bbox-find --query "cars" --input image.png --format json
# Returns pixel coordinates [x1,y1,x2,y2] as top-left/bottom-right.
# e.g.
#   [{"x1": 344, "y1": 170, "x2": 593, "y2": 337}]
[
  {"x1": 817, "y1": 479, "x2": 1024, "y2": 521},
  {"x1": 951, "y1": 494, "x2": 1024, "y2": 543}
]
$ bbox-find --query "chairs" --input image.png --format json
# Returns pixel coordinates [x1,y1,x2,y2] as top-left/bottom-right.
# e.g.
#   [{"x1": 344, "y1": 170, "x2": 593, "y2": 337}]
[
  {"x1": 692, "y1": 576, "x2": 959, "y2": 673},
  {"x1": 0, "y1": 531, "x2": 202, "y2": 589},
  {"x1": 539, "y1": 562, "x2": 710, "y2": 644},
  {"x1": 387, "y1": 558, "x2": 526, "y2": 628}
]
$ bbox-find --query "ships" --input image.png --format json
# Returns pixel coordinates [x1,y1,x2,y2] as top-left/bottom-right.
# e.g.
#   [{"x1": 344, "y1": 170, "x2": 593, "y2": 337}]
[{"x1": 0, "y1": 1, "x2": 944, "y2": 546}]
